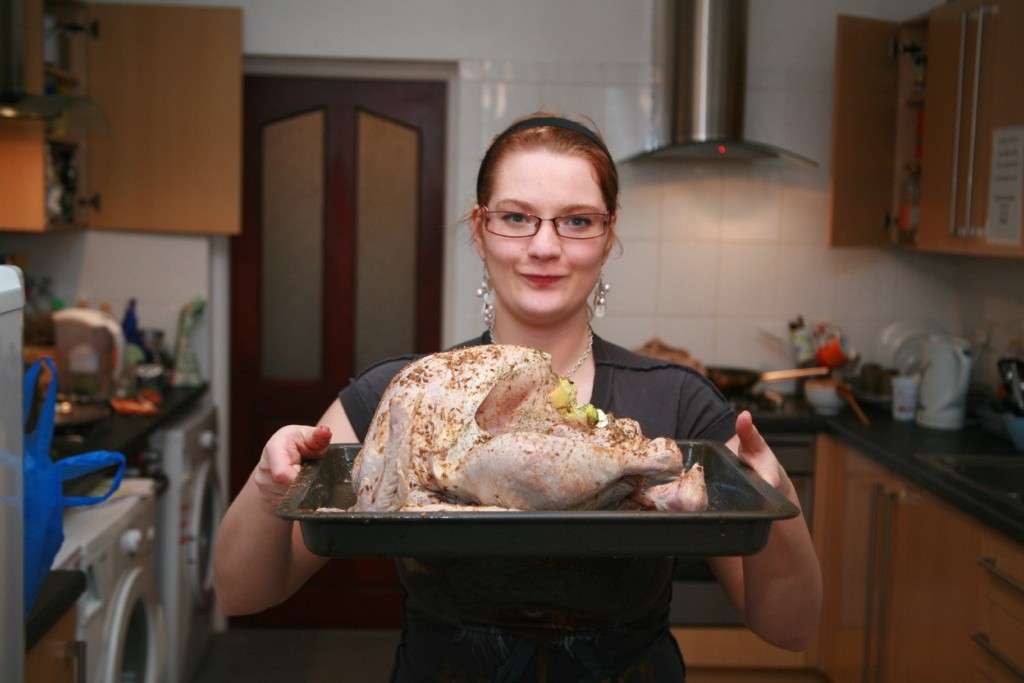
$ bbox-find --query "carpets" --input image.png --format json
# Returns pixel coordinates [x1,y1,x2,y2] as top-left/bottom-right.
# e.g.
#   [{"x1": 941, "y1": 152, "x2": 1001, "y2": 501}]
[
  {"x1": 193, "y1": 628, "x2": 403, "y2": 682},
  {"x1": 687, "y1": 665, "x2": 831, "y2": 683}
]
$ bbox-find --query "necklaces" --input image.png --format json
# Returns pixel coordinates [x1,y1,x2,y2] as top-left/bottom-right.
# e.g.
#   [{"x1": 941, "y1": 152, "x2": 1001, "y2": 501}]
[{"x1": 486, "y1": 321, "x2": 594, "y2": 381}]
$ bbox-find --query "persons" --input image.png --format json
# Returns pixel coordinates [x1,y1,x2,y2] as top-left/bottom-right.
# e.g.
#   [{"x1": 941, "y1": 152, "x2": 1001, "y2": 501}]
[{"x1": 210, "y1": 111, "x2": 823, "y2": 683}]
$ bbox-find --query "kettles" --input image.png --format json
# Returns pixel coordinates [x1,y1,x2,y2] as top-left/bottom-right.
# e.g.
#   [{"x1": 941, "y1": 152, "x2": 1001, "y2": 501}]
[{"x1": 53, "y1": 309, "x2": 124, "y2": 394}]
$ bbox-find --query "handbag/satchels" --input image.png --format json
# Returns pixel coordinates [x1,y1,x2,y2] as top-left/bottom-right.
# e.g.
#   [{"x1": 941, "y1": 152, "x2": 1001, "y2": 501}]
[{"x1": 23, "y1": 354, "x2": 127, "y2": 635}]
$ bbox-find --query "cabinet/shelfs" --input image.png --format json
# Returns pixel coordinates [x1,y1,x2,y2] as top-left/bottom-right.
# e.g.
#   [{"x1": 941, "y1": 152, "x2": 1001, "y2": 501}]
[
  {"x1": 829, "y1": 13, "x2": 932, "y2": 253},
  {"x1": 804, "y1": 430, "x2": 893, "y2": 683},
  {"x1": 24, "y1": 571, "x2": 89, "y2": 683},
  {"x1": 884, "y1": 472, "x2": 1023, "y2": 683},
  {"x1": 915, "y1": 1, "x2": 1023, "y2": 258},
  {"x1": 1, "y1": 0, "x2": 246, "y2": 237}
]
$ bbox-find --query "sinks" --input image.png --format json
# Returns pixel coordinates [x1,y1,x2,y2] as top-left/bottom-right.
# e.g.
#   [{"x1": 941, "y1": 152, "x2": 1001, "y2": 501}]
[{"x1": 915, "y1": 437, "x2": 1024, "y2": 510}]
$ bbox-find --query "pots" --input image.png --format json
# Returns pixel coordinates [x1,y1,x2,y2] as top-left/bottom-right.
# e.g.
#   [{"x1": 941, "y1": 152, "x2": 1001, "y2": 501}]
[{"x1": 706, "y1": 360, "x2": 828, "y2": 390}]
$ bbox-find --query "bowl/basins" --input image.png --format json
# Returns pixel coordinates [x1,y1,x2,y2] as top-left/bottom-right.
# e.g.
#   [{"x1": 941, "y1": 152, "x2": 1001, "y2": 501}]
[{"x1": 805, "y1": 380, "x2": 853, "y2": 414}]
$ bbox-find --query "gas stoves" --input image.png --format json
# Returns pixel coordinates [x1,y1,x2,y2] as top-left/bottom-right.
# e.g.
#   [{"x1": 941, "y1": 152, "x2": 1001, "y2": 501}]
[{"x1": 702, "y1": 377, "x2": 827, "y2": 431}]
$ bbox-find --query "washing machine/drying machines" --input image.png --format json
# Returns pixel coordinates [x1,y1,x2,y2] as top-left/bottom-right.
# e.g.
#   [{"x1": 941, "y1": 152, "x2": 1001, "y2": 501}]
[
  {"x1": 140, "y1": 395, "x2": 223, "y2": 683},
  {"x1": 48, "y1": 473, "x2": 169, "y2": 683}
]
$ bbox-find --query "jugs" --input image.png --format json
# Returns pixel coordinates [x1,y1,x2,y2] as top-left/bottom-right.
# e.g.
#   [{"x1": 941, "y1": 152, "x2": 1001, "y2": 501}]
[{"x1": 912, "y1": 331, "x2": 973, "y2": 431}]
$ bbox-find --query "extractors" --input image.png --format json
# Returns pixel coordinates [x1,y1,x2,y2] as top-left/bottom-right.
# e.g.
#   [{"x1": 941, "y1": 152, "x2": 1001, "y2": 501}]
[{"x1": 599, "y1": 1, "x2": 835, "y2": 176}]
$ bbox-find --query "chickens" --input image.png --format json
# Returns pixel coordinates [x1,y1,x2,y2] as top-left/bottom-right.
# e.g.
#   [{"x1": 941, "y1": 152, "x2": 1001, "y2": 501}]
[{"x1": 313, "y1": 342, "x2": 708, "y2": 514}]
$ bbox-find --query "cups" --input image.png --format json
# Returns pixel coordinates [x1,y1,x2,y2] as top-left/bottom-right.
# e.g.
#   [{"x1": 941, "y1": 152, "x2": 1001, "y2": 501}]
[{"x1": 893, "y1": 373, "x2": 920, "y2": 421}]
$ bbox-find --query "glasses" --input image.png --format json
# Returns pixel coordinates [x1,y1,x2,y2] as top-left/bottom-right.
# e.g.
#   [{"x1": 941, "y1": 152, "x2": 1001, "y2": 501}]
[{"x1": 478, "y1": 205, "x2": 613, "y2": 241}]
